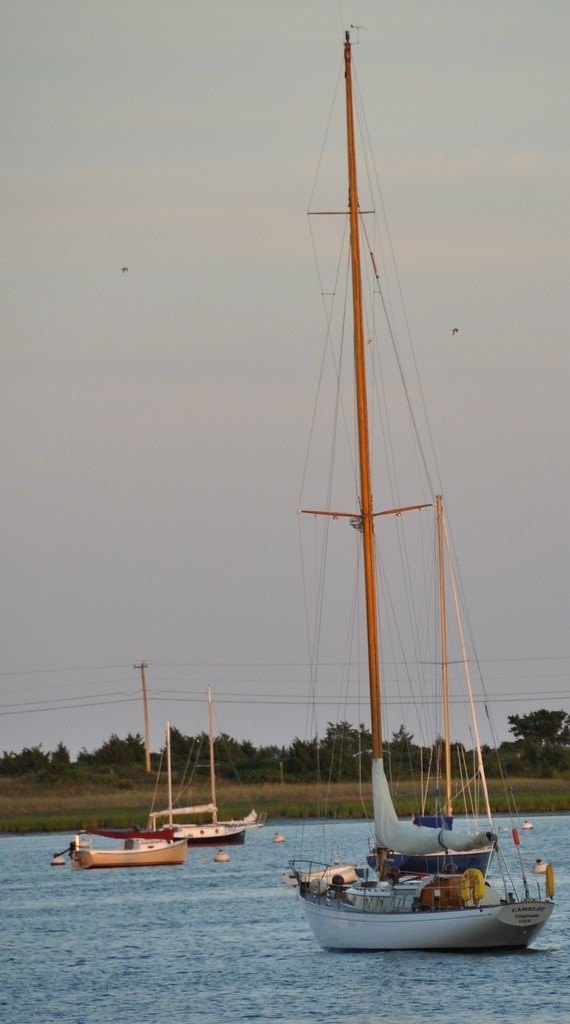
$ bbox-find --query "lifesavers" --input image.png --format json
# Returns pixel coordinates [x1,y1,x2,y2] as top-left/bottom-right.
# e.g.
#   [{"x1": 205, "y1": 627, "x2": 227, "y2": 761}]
[{"x1": 460, "y1": 867, "x2": 485, "y2": 901}]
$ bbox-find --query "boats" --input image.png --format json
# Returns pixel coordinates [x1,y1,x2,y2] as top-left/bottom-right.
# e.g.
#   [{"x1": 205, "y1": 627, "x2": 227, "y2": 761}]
[{"x1": 69, "y1": 720, "x2": 188, "y2": 870}]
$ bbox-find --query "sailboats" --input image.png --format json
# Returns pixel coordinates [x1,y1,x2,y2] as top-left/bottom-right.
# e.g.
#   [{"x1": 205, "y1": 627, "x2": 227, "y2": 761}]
[
  {"x1": 288, "y1": 25, "x2": 555, "y2": 952},
  {"x1": 133, "y1": 682, "x2": 268, "y2": 847}
]
